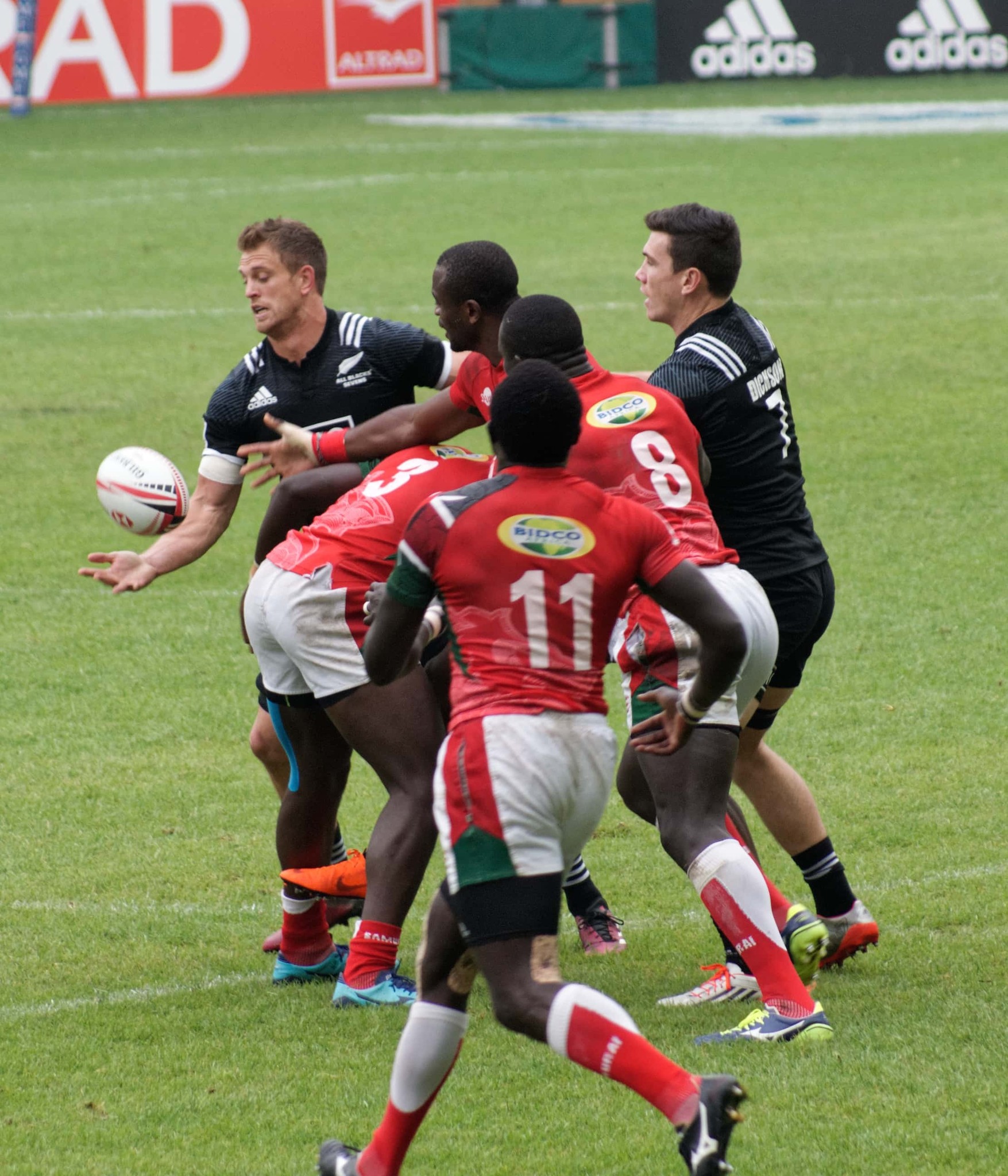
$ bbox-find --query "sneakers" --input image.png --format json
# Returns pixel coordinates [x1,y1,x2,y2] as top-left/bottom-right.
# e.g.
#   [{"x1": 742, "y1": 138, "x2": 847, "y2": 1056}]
[
  {"x1": 779, "y1": 903, "x2": 830, "y2": 988},
  {"x1": 262, "y1": 893, "x2": 363, "y2": 953},
  {"x1": 574, "y1": 897, "x2": 627, "y2": 957},
  {"x1": 271, "y1": 944, "x2": 349, "y2": 984},
  {"x1": 694, "y1": 1000, "x2": 836, "y2": 1047},
  {"x1": 316, "y1": 1139, "x2": 360, "y2": 1176},
  {"x1": 278, "y1": 848, "x2": 366, "y2": 899},
  {"x1": 328, "y1": 959, "x2": 417, "y2": 1009},
  {"x1": 656, "y1": 962, "x2": 763, "y2": 1008},
  {"x1": 678, "y1": 1074, "x2": 747, "y2": 1176},
  {"x1": 817, "y1": 898, "x2": 879, "y2": 969}
]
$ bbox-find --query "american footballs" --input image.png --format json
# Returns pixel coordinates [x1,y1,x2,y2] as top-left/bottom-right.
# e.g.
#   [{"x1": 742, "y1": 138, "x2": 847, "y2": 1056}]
[{"x1": 95, "y1": 445, "x2": 190, "y2": 537}]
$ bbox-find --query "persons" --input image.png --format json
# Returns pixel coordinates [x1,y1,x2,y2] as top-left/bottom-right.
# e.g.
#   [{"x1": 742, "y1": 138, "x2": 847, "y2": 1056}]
[
  {"x1": 77, "y1": 202, "x2": 877, "y2": 1046},
  {"x1": 313, "y1": 368, "x2": 748, "y2": 1176}
]
